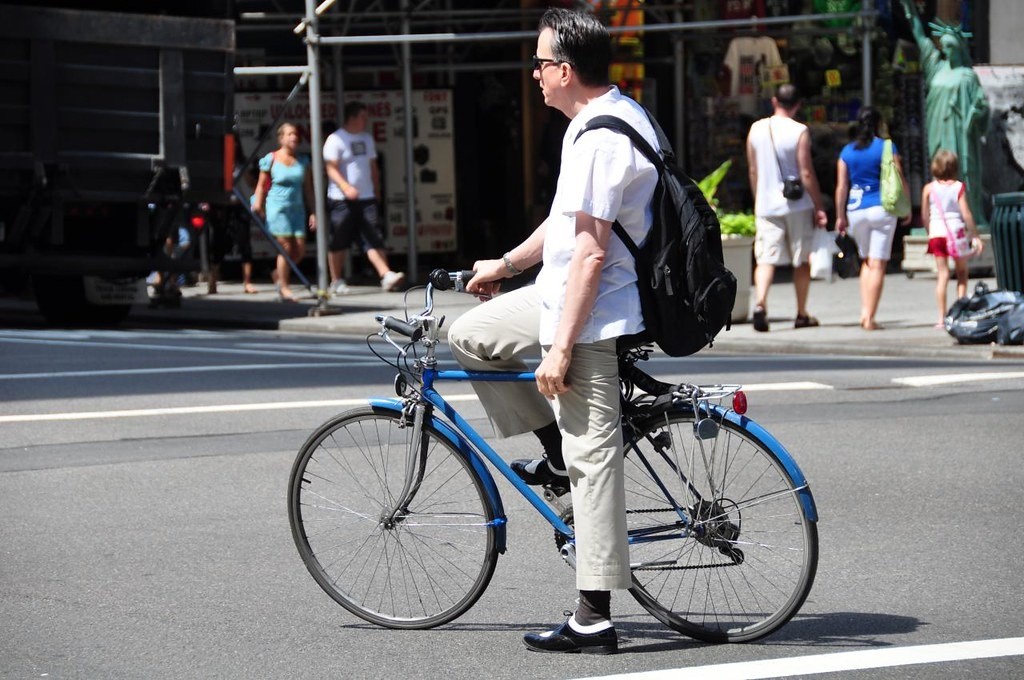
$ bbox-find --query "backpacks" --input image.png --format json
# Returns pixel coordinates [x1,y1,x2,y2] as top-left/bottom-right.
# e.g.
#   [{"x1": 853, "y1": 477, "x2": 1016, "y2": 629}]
[{"x1": 571, "y1": 98, "x2": 737, "y2": 357}]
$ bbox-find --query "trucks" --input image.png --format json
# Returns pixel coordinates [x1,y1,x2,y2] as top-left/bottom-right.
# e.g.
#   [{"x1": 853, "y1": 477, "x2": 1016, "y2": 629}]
[{"x1": 1, "y1": 6, "x2": 236, "y2": 328}]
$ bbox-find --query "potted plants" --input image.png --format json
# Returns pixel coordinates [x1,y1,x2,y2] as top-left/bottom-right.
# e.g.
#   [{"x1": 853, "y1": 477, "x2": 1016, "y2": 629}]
[{"x1": 689, "y1": 161, "x2": 757, "y2": 322}]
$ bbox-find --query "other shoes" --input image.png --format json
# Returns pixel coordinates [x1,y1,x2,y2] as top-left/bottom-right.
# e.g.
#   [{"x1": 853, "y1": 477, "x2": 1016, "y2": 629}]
[
  {"x1": 752, "y1": 307, "x2": 769, "y2": 332},
  {"x1": 794, "y1": 312, "x2": 819, "y2": 328}
]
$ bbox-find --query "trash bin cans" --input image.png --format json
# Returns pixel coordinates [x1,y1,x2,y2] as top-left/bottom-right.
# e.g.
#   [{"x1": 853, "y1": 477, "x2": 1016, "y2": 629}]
[{"x1": 990, "y1": 192, "x2": 1024, "y2": 296}]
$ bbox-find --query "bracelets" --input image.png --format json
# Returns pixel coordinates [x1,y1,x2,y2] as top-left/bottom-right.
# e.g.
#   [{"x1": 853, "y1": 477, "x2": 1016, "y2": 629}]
[
  {"x1": 503, "y1": 252, "x2": 523, "y2": 275},
  {"x1": 341, "y1": 183, "x2": 349, "y2": 190}
]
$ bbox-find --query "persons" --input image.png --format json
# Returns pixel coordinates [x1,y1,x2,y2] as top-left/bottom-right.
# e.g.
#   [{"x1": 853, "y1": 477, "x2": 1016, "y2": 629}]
[
  {"x1": 835, "y1": 107, "x2": 912, "y2": 330},
  {"x1": 323, "y1": 103, "x2": 405, "y2": 296},
  {"x1": 206, "y1": 214, "x2": 258, "y2": 294},
  {"x1": 253, "y1": 123, "x2": 316, "y2": 302},
  {"x1": 145, "y1": 227, "x2": 190, "y2": 289},
  {"x1": 747, "y1": 83, "x2": 828, "y2": 331},
  {"x1": 922, "y1": 150, "x2": 985, "y2": 328},
  {"x1": 447, "y1": 9, "x2": 662, "y2": 653},
  {"x1": 902, "y1": 0, "x2": 989, "y2": 225}
]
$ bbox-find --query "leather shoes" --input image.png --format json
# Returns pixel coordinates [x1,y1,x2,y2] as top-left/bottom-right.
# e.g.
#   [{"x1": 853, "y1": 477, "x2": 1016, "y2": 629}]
[
  {"x1": 521, "y1": 607, "x2": 619, "y2": 655},
  {"x1": 510, "y1": 453, "x2": 571, "y2": 492}
]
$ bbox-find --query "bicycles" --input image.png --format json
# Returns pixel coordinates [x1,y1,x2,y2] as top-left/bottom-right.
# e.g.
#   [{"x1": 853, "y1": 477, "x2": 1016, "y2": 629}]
[{"x1": 286, "y1": 269, "x2": 818, "y2": 644}]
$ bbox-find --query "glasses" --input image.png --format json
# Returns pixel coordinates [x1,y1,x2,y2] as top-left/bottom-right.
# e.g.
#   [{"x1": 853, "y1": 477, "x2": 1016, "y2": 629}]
[{"x1": 532, "y1": 55, "x2": 572, "y2": 70}]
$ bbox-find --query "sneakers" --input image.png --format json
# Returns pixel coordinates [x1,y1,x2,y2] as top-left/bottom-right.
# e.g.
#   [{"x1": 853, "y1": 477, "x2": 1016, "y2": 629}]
[
  {"x1": 329, "y1": 279, "x2": 352, "y2": 296},
  {"x1": 379, "y1": 271, "x2": 404, "y2": 292}
]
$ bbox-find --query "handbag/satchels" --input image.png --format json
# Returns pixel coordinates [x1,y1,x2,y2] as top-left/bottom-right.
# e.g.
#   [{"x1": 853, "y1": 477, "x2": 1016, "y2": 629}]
[
  {"x1": 262, "y1": 150, "x2": 278, "y2": 196},
  {"x1": 832, "y1": 231, "x2": 862, "y2": 279},
  {"x1": 946, "y1": 223, "x2": 977, "y2": 258},
  {"x1": 782, "y1": 176, "x2": 805, "y2": 200},
  {"x1": 879, "y1": 139, "x2": 912, "y2": 217}
]
